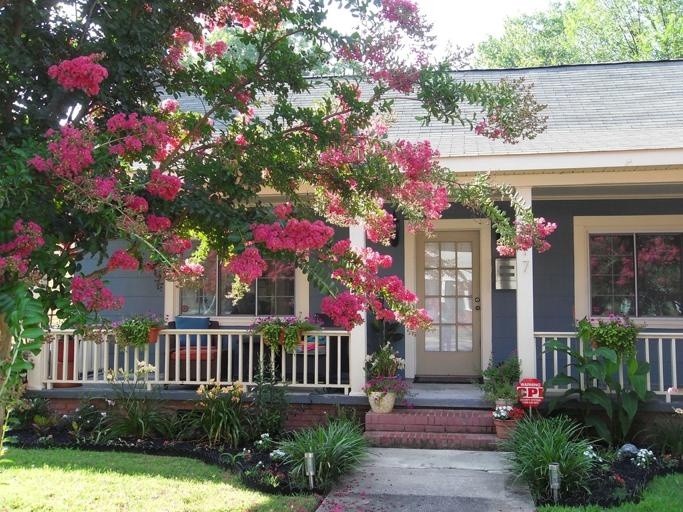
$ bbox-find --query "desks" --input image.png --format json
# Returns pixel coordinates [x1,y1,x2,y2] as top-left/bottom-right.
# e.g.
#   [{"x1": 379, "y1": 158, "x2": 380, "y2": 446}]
[{"x1": 236, "y1": 337, "x2": 282, "y2": 381}]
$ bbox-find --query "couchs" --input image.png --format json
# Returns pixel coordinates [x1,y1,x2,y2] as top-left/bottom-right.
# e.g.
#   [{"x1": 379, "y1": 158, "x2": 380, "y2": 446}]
[
  {"x1": 167, "y1": 317, "x2": 234, "y2": 381},
  {"x1": 281, "y1": 313, "x2": 345, "y2": 381}
]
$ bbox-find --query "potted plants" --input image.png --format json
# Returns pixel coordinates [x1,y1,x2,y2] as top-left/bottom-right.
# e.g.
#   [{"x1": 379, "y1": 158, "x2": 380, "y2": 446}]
[
  {"x1": 469, "y1": 350, "x2": 526, "y2": 439},
  {"x1": 362, "y1": 341, "x2": 419, "y2": 417}
]
